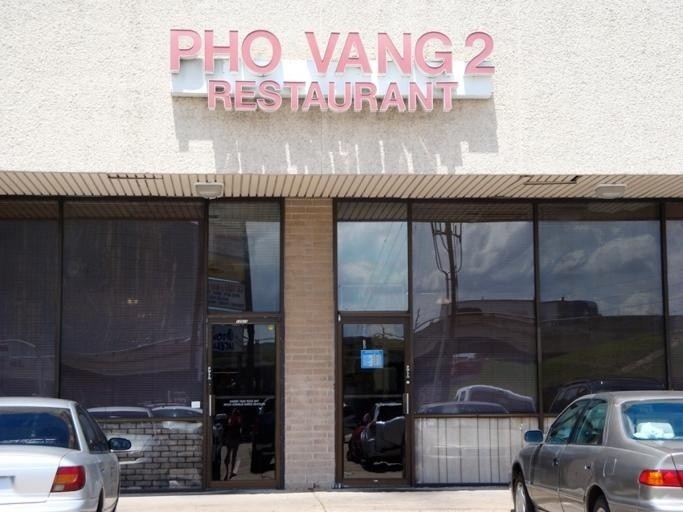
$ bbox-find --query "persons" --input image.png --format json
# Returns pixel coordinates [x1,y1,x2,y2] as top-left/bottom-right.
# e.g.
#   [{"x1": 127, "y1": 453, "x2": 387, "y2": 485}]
[{"x1": 219, "y1": 407, "x2": 243, "y2": 481}]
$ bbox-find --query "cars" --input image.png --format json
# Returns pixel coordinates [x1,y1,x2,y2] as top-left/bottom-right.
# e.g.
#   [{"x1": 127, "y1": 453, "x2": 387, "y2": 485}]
[
  {"x1": 88, "y1": 405, "x2": 229, "y2": 490},
  {"x1": 416, "y1": 385, "x2": 536, "y2": 415},
  {"x1": 511, "y1": 390, "x2": 683, "y2": 512},
  {"x1": 549, "y1": 377, "x2": 665, "y2": 413},
  {"x1": 347, "y1": 403, "x2": 405, "y2": 473},
  {"x1": 0, "y1": 396, "x2": 131, "y2": 512}
]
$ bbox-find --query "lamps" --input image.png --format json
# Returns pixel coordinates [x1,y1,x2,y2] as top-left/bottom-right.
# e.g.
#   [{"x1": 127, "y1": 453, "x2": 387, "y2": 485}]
[
  {"x1": 593, "y1": 184, "x2": 626, "y2": 198},
  {"x1": 194, "y1": 182, "x2": 223, "y2": 198}
]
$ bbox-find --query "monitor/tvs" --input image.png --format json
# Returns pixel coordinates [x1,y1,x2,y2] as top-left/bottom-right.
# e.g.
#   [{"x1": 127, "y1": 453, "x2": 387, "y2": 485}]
[{"x1": 361, "y1": 350, "x2": 384, "y2": 369}]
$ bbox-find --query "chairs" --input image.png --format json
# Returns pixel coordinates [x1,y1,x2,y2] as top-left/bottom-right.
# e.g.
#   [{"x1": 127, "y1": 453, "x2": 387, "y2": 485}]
[{"x1": 636, "y1": 420, "x2": 675, "y2": 438}]
